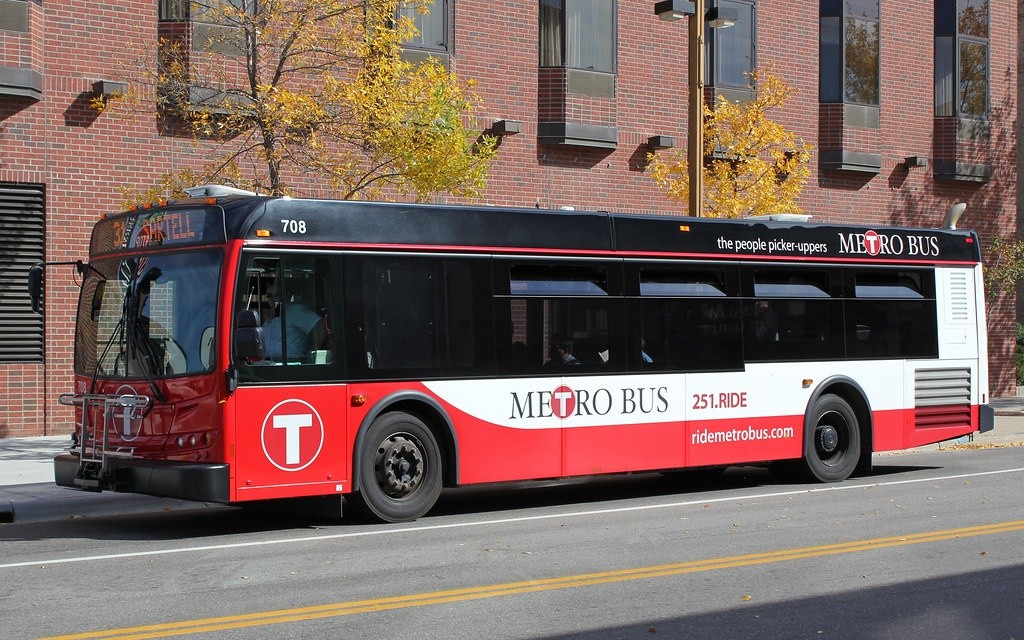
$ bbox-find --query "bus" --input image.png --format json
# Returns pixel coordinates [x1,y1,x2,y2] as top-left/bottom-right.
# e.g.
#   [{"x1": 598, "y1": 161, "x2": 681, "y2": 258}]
[{"x1": 25, "y1": 184, "x2": 995, "y2": 522}]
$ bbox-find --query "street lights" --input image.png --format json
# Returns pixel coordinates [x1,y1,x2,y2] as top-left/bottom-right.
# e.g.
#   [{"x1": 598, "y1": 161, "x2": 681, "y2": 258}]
[{"x1": 653, "y1": 1, "x2": 706, "y2": 218}]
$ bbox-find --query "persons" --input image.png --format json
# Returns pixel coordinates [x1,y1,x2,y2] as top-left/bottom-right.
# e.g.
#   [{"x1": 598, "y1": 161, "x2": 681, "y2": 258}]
[
  {"x1": 255, "y1": 277, "x2": 327, "y2": 364},
  {"x1": 512, "y1": 335, "x2": 653, "y2": 368}
]
